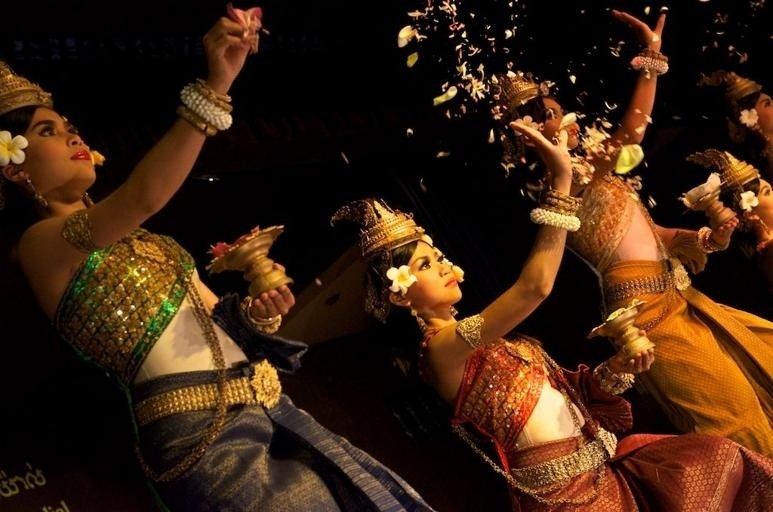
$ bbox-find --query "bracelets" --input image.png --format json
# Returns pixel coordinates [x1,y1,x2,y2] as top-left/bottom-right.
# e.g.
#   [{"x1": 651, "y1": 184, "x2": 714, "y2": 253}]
[
  {"x1": 694, "y1": 226, "x2": 731, "y2": 255},
  {"x1": 627, "y1": 47, "x2": 670, "y2": 77},
  {"x1": 237, "y1": 297, "x2": 282, "y2": 336},
  {"x1": 195, "y1": 77, "x2": 233, "y2": 103},
  {"x1": 528, "y1": 188, "x2": 582, "y2": 234},
  {"x1": 175, "y1": 104, "x2": 217, "y2": 137},
  {"x1": 190, "y1": 83, "x2": 232, "y2": 113},
  {"x1": 591, "y1": 359, "x2": 636, "y2": 397},
  {"x1": 178, "y1": 87, "x2": 232, "y2": 132}
]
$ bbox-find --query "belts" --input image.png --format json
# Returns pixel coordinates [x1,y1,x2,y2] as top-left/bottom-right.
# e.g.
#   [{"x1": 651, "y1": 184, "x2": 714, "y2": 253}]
[
  {"x1": 510, "y1": 427, "x2": 618, "y2": 488},
  {"x1": 605, "y1": 265, "x2": 691, "y2": 303},
  {"x1": 132, "y1": 359, "x2": 282, "y2": 429}
]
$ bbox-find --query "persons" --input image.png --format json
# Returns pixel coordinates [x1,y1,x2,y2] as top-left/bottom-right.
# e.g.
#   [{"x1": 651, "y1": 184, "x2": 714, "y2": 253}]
[
  {"x1": 725, "y1": 94, "x2": 773, "y2": 167},
  {"x1": 722, "y1": 177, "x2": 773, "y2": 279},
  {"x1": 499, "y1": 9, "x2": 773, "y2": 464},
  {"x1": 363, "y1": 121, "x2": 773, "y2": 512},
  {"x1": 0, "y1": 16, "x2": 436, "y2": 510}
]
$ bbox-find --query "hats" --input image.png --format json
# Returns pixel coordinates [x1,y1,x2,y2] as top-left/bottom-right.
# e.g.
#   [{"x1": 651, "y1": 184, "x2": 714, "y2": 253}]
[
  {"x1": 329, "y1": 197, "x2": 424, "y2": 260},
  {"x1": 0, "y1": 61, "x2": 54, "y2": 115},
  {"x1": 685, "y1": 148, "x2": 760, "y2": 192},
  {"x1": 695, "y1": 69, "x2": 762, "y2": 102},
  {"x1": 490, "y1": 74, "x2": 539, "y2": 110}
]
